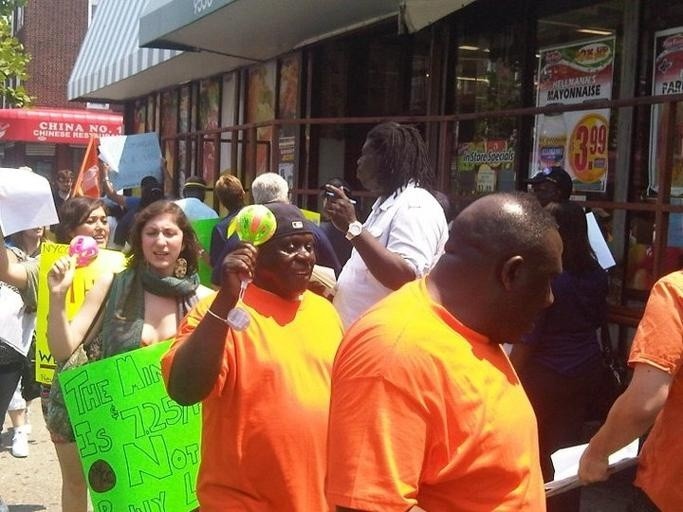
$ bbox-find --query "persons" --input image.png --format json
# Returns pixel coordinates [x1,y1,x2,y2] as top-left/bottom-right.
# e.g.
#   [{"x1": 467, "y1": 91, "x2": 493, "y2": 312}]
[{"x1": 0, "y1": 120, "x2": 683, "y2": 511}]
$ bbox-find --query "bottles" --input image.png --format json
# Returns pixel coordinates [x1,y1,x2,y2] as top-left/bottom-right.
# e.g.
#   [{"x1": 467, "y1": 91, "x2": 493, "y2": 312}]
[{"x1": 539, "y1": 104, "x2": 565, "y2": 173}]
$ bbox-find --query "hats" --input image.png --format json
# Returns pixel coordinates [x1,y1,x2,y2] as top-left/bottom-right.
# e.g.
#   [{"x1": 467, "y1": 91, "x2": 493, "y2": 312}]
[
  {"x1": 522, "y1": 165, "x2": 572, "y2": 192},
  {"x1": 182, "y1": 177, "x2": 206, "y2": 197},
  {"x1": 262, "y1": 202, "x2": 316, "y2": 240}
]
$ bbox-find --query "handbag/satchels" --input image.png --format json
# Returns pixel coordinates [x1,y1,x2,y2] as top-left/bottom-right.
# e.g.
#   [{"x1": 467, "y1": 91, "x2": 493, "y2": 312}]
[
  {"x1": 581, "y1": 368, "x2": 632, "y2": 422},
  {"x1": 49, "y1": 344, "x2": 89, "y2": 406}
]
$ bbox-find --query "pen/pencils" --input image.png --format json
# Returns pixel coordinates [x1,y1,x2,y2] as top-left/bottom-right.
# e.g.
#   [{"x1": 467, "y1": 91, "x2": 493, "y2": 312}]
[{"x1": 326, "y1": 192, "x2": 357, "y2": 204}]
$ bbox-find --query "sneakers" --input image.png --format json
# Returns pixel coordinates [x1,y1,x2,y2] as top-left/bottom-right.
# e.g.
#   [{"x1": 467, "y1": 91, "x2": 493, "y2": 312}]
[{"x1": 11, "y1": 428, "x2": 29, "y2": 457}]
